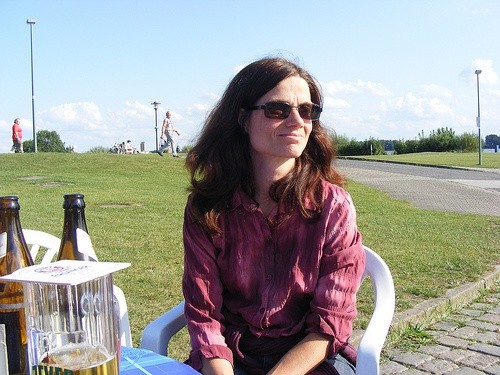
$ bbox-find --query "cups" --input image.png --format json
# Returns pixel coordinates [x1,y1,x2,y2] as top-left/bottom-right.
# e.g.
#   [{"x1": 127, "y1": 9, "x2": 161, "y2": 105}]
[{"x1": 22, "y1": 274, "x2": 119, "y2": 375}]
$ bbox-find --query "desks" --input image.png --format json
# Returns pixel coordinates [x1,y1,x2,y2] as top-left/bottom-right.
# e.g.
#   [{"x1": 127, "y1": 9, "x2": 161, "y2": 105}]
[{"x1": 120, "y1": 346, "x2": 202, "y2": 375}]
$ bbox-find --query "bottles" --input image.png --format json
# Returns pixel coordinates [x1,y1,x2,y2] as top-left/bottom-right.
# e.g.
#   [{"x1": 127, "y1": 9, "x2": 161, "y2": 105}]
[
  {"x1": 55, "y1": 193, "x2": 99, "y2": 261},
  {"x1": 0, "y1": 196, "x2": 35, "y2": 375}
]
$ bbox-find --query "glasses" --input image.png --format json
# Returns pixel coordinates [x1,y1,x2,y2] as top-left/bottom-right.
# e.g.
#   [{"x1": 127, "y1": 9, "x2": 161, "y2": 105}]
[{"x1": 250, "y1": 102, "x2": 323, "y2": 120}]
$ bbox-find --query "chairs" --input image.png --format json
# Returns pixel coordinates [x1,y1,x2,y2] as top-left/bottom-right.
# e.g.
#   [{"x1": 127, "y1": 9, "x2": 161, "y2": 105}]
[
  {"x1": 142, "y1": 245, "x2": 395, "y2": 375},
  {"x1": 0, "y1": 230, "x2": 132, "y2": 348}
]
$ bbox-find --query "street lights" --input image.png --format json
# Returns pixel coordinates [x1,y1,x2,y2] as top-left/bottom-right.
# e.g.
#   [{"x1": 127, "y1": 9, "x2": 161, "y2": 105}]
[
  {"x1": 26, "y1": 17, "x2": 38, "y2": 153},
  {"x1": 149, "y1": 101, "x2": 162, "y2": 153},
  {"x1": 475, "y1": 69, "x2": 483, "y2": 166}
]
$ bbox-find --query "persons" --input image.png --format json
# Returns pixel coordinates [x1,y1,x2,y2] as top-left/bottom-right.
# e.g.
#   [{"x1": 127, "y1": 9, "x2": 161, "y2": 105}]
[
  {"x1": 158, "y1": 110, "x2": 180, "y2": 157},
  {"x1": 10, "y1": 118, "x2": 24, "y2": 153},
  {"x1": 182, "y1": 57, "x2": 367, "y2": 375}
]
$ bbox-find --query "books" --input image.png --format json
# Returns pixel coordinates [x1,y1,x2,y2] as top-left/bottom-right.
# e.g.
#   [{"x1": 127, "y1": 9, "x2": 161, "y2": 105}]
[{"x1": 0, "y1": 260, "x2": 131, "y2": 285}]
